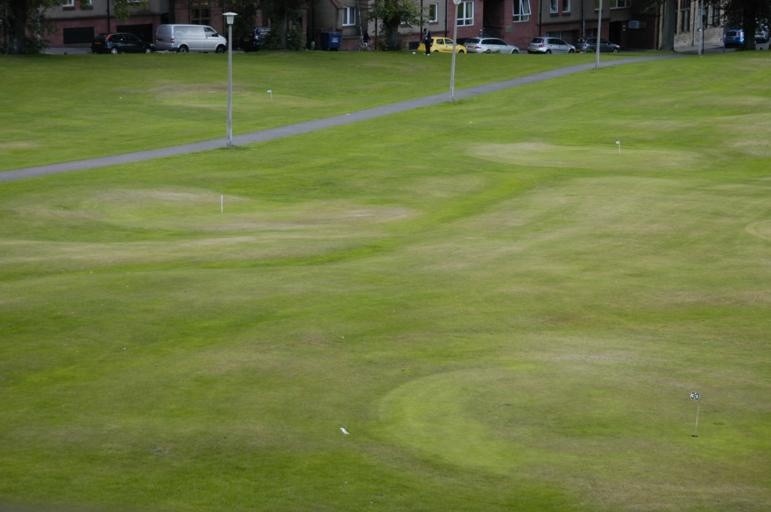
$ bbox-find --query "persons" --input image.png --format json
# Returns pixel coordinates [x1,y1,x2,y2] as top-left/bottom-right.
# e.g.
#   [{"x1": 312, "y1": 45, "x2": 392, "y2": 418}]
[{"x1": 424, "y1": 28, "x2": 431, "y2": 56}]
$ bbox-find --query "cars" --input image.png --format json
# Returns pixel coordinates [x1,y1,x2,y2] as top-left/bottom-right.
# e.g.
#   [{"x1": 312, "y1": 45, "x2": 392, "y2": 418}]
[
  {"x1": 575, "y1": 38, "x2": 622, "y2": 54},
  {"x1": 463, "y1": 37, "x2": 521, "y2": 54},
  {"x1": 755, "y1": 23, "x2": 770, "y2": 43},
  {"x1": 528, "y1": 36, "x2": 576, "y2": 55},
  {"x1": 419, "y1": 36, "x2": 467, "y2": 55},
  {"x1": 92, "y1": 32, "x2": 155, "y2": 56}
]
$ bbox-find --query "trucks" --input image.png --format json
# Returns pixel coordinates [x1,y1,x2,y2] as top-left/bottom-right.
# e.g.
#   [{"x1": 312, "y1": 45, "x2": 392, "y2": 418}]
[{"x1": 724, "y1": 22, "x2": 745, "y2": 48}]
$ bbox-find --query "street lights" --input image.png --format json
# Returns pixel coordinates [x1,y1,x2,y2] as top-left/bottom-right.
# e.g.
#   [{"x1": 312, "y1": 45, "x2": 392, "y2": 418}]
[
  {"x1": 450, "y1": 0, "x2": 462, "y2": 98},
  {"x1": 222, "y1": 11, "x2": 239, "y2": 147}
]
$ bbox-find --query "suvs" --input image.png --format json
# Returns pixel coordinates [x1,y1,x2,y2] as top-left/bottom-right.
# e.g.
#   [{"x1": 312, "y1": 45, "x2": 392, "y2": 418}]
[
  {"x1": 156, "y1": 23, "x2": 228, "y2": 54},
  {"x1": 240, "y1": 26, "x2": 292, "y2": 51}
]
